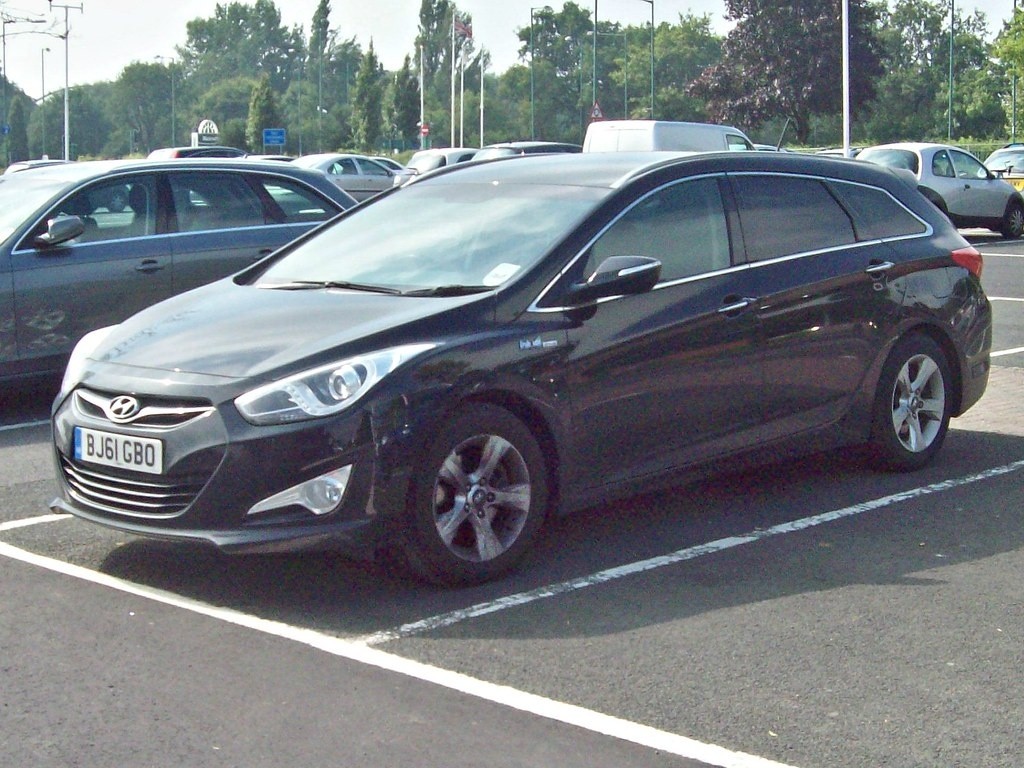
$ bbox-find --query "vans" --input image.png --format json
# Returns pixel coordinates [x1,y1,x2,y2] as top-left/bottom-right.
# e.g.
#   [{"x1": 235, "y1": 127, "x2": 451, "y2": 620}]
[{"x1": 579, "y1": 119, "x2": 758, "y2": 153}]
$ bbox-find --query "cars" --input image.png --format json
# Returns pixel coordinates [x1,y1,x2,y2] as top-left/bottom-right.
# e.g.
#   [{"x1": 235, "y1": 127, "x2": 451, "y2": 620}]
[
  {"x1": 855, "y1": 142, "x2": 1024, "y2": 241},
  {"x1": 815, "y1": 146, "x2": 868, "y2": 158},
  {"x1": 0, "y1": 137, "x2": 585, "y2": 230},
  {"x1": 731, "y1": 143, "x2": 786, "y2": 153},
  {"x1": 0, "y1": 157, "x2": 363, "y2": 388},
  {"x1": 42, "y1": 149, "x2": 995, "y2": 590},
  {"x1": 977, "y1": 142, "x2": 1024, "y2": 199}
]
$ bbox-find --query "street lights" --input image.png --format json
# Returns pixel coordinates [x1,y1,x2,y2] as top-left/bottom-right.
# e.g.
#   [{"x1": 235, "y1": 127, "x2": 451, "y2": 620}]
[
  {"x1": 2, "y1": 19, "x2": 48, "y2": 167},
  {"x1": 641, "y1": 0, "x2": 654, "y2": 119},
  {"x1": 530, "y1": 5, "x2": 550, "y2": 141},
  {"x1": 586, "y1": 29, "x2": 629, "y2": 120},
  {"x1": 154, "y1": 54, "x2": 175, "y2": 148},
  {"x1": 40, "y1": 47, "x2": 51, "y2": 159}
]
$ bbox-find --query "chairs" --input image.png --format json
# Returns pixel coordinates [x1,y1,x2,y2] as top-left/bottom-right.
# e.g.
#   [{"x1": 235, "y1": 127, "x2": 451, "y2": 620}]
[
  {"x1": 67, "y1": 191, "x2": 100, "y2": 243},
  {"x1": 129, "y1": 185, "x2": 147, "y2": 236}
]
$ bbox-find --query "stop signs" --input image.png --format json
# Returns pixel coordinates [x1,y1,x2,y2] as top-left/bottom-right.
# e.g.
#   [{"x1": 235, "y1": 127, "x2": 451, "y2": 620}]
[{"x1": 421, "y1": 124, "x2": 429, "y2": 136}]
